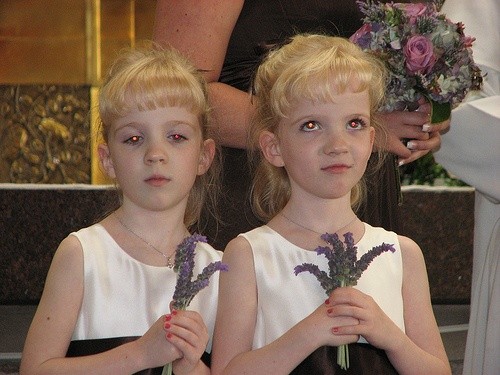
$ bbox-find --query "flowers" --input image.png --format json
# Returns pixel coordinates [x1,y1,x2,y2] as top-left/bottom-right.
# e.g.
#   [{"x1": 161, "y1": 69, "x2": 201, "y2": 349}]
[
  {"x1": 347, "y1": 0, "x2": 488, "y2": 207},
  {"x1": 292, "y1": 232, "x2": 397, "y2": 371},
  {"x1": 161, "y1": 232, "x2": 230, "y2": 375}
]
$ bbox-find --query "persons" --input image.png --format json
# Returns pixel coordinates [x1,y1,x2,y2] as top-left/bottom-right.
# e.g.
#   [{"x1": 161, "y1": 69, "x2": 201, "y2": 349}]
[
  {"x1": 20, "y1": 40, "x2": 225, "y2": 375},
  {"x1": 154, "y1": 0, "x2": 451, "y2": 251},
  {"x1": 210, "y1": 33, "x2": 452, "y2": 375}
]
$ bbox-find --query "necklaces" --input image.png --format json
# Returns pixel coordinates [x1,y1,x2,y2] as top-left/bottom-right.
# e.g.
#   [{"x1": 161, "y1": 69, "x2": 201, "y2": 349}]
[
  {"x1": 113, "y1": 213, "x2": 188, "y2": 269},
  {"x1": 281, "y1": 214, "x2": 358, "y2": 245}
]
